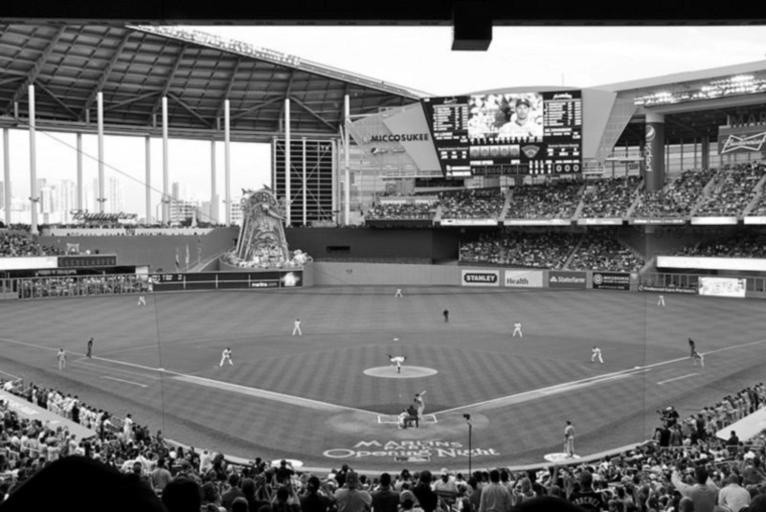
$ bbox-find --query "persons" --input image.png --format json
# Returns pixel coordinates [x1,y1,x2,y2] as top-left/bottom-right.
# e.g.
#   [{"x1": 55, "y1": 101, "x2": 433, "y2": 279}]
[
  {"x1": 86, "y1": 337, "x2": 94, "y2": 358},
  {"x1": 370, "y1": 161, "x2": 766, "y2": 218},
  {"x1": 512, "y1": 321, "x2": 523, "y2": 338},
  {"x1": 1, "y1": 377, "x2": 766, "y2": 512},
  {"x1": 498, "y1": 97, "x2": 543, "y2": 138},
  {"x1": 57, "y1": 347, "x2": 68, "y2": 371},
  {"x1": 687, "y1": 336, "x2": 696, "y2": 357},
  {"x1": 292, "y1": 318, "x2": 302, "y2": 337},
  {"x1": 388, "y1": 353, "x2": 407, "y2": 373},
  {"x1": 590, "y1": 345, "x2": 604, "y2": 364},
  {"x1": 219, "y1": 347, "x2": 233, "y2": 367},
  {"x1": 393, "y1": 287, "x2": 403, "y2": 298},
  {"x1": 693, "y1": 350, "x2": 704, "y2": 367},
  {"x1": 442, "y1": 308, "x2": 449, "y2": 322},
  {"x1": 1, "y1": 207, "x2": 159, "y2": 307},
  {"x1": 463, "y1": 228, "x2": 766, "y2": 269},
  {"x1": 657, "y1": 294, "x2": 666, "y2": 307}
]
